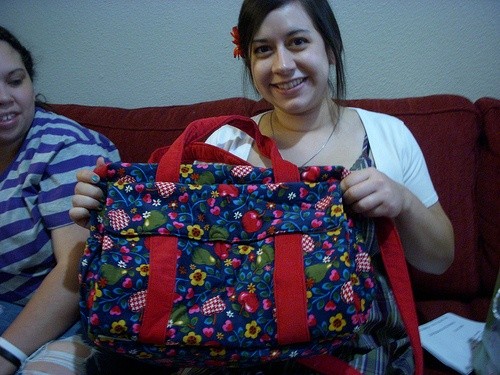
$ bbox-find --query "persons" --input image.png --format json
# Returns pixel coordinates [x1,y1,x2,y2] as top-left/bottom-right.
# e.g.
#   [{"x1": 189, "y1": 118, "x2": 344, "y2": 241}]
[
  {"x1": 0, "y1": 23, "x2": 122, "y2": 375},
  {"x1": 68, "y1": 0, "x2": 458, "y2": 375}
]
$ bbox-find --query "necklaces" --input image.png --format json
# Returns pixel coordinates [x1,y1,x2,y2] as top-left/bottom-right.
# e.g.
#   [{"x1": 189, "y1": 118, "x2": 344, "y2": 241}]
[{"x1": 268, "y1": 106, "x2": 344, "y2": 168}]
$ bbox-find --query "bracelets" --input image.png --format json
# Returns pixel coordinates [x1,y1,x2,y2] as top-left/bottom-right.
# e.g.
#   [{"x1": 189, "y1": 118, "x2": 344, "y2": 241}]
[
  {"x1": 0, "y1": 336, "x2": 29, "y2": 363},
  {"x1": 0, "y1": 346, "x2": 23, "y2": 368}
]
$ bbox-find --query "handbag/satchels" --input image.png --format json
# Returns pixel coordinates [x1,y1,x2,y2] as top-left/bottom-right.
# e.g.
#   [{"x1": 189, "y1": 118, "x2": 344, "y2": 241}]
[{"x1": 79, "y1": 116, "x2": 380, "y2": 363}]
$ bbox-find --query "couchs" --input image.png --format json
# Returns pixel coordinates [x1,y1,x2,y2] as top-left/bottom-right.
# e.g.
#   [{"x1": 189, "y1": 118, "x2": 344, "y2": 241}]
[{"x1": 34, "y1": 94, "x2": 499, "y2": 375}]
